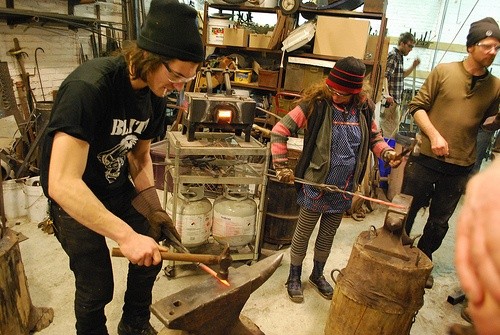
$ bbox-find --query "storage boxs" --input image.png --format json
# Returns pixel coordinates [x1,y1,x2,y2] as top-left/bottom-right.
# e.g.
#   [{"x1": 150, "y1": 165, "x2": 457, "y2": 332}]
[{"x1": 194, "y1": 0, "x2": 390, "y2": 172}]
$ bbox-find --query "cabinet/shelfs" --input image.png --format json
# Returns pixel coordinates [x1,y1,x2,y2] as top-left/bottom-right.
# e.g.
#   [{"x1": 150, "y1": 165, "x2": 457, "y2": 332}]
[
  {"x1": 0, "y1": 0, "x2": 100, "y2": 32},
  {"x1": 388, "y1": 34, "x2": 474, "y2": 80}
]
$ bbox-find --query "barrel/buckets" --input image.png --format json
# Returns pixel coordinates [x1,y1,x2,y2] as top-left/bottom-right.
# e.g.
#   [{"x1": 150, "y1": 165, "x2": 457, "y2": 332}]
[
  {"x1": 1, "y1": 176, "x2": 28, "y2": 220},
  {"x1": 207, "y1": 18, "x2": 229, "y2": 45},
  {"x1": 22, "y1": 176, "x2": 49, "y2": 223}
]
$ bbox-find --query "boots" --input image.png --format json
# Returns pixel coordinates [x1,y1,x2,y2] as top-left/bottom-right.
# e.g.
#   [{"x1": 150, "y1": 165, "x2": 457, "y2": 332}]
[
  {"x1": 286, "y1": 263, "x2": 304, "y2": 302},
  {"x1": 309, "y1": 258, "x2": 334, "y2": 300}
]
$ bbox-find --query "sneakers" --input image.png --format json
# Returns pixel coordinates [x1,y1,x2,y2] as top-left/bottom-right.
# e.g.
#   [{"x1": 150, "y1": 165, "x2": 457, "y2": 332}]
[
  {"x1": 425, "y1": 275, "x2": 433, "y2": 288},
  {"x1": 117, "y1": 317, "x2": 158, "y2": 335}
]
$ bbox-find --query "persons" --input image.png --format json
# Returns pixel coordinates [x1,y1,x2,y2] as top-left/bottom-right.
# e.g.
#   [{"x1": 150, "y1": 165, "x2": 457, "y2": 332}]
[
  {"x1": 377, "y1": 31, "x2": 421, "y2": 193},
  {"x1": 41, "y1": 3, "x2": 205, "y2": 335},
  {"x1": 404, "y1": 18, "x2": 499, "y2": 261},
  {"x1": 266, "y1": 56, "x2": 403, "y2": 303},
  {"x1": 454, "y1": 153, "x2": 499, "y2": 335}
]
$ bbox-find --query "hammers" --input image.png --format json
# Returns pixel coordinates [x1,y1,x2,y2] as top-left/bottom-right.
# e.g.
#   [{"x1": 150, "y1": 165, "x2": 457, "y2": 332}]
[
  {"x1": 382, "y1": 135, "x2": 423, "y2": 169},
  {"x1": 111, "y1": 242, "x2": 231, "y2": 281}
]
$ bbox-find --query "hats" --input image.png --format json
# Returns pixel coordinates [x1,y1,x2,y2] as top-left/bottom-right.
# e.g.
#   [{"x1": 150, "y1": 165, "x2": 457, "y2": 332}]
[
  {"x1": 326, "y1": 56, "x2": 366, "y2": 94},
  {"x1": 467, "y1": 17, "x2": 500, "y2": 46},
  {"x1": 135, "y1": 0, "x2": 205, "y2": 63}
]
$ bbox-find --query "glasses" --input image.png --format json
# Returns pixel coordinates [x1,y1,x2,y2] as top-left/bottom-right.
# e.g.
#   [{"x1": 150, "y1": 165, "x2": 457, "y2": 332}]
[
  {"x1": 163, "y1": 60, "x2": 196, "y2": 83},
  {"x1": 405, "y1": 42, "x2": 414, "y2": 48},
  {"x1": 477, "y1": 43, "x2": 500, "y2": 52},
  {"x1": 326, "y1": 84, "x2": 350, "y2": 96}
]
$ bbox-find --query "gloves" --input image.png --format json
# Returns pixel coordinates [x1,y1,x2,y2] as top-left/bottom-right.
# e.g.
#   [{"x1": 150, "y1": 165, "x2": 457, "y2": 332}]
[
  {"x1": 275, "y1": 168, "x2": 294, "y2": 182},
  {"x1": 130, "y1": 186, "x2": 182, "y2": 246},
  {"x1": 383, "y1": 150, "x2": 403, "y2": 169}
]
